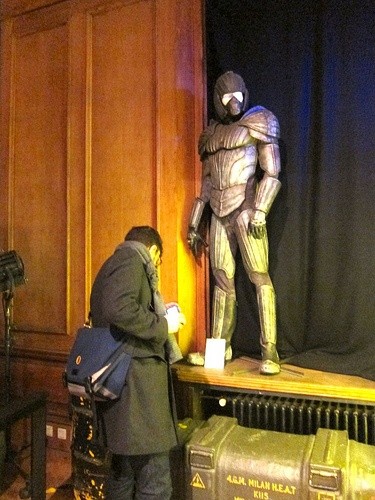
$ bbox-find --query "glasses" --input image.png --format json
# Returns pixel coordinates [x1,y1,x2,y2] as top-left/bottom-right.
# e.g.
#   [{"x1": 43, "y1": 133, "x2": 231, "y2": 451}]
[{"x1": 153, "y1": 251, "x2": 163, "y2": 269}]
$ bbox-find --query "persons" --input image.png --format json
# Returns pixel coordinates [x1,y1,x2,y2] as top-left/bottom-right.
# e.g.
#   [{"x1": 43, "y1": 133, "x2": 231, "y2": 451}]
[
  {"x1": 186, "y1": 70, "x2": 282, "y2": 374},
  {"x1": 90, "y1": 225, "x2": 184, "y2": 500}
]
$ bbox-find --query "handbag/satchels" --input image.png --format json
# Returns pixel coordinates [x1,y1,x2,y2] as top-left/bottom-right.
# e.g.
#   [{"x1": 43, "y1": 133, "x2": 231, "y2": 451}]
[{"x1": 65, "y1": 324, "x2": 134, "y2": 401}]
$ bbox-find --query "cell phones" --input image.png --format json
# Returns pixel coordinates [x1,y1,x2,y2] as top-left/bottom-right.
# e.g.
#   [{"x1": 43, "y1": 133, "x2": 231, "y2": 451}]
[{"x1": 166, "y1": 306, "x2": 179, "y2": 315}]
